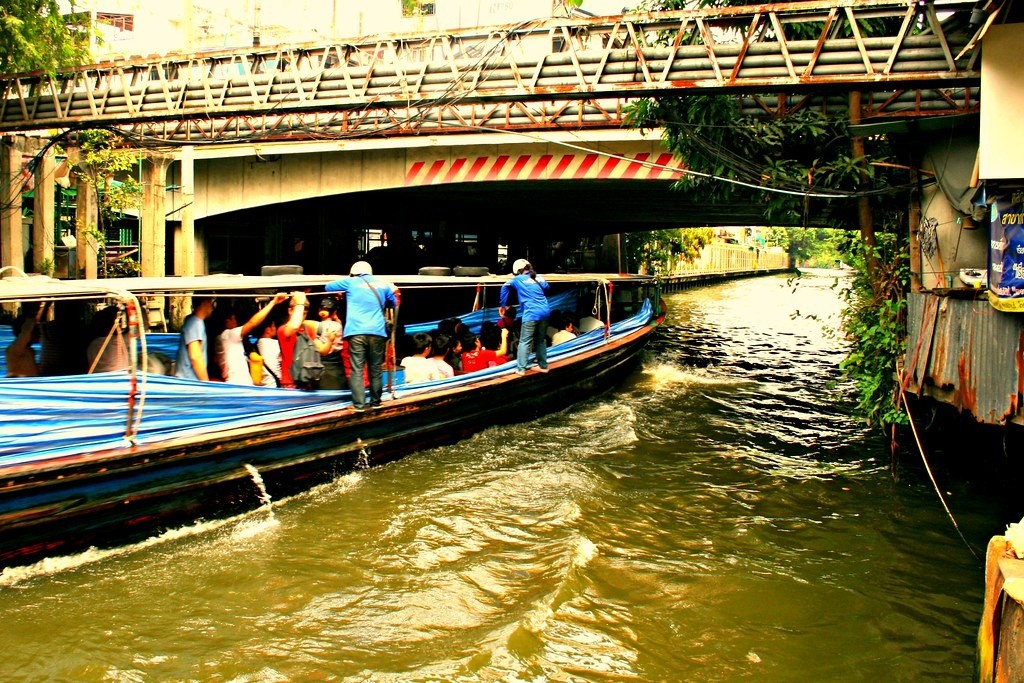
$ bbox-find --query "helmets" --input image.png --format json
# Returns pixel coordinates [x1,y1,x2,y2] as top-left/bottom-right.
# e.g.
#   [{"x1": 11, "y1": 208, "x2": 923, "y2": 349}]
[
  {"x1": 349, "y1": 261, "x2": 373, "y2": 276},
  {"x1": 513, "y1": 259, "x2": 532, "y2": 276}
]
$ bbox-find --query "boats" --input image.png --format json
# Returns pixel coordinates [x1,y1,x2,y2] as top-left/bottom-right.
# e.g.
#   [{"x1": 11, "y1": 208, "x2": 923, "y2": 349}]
[{"x1": 0, "y1": 265, "x2": 670, "y2": 574}]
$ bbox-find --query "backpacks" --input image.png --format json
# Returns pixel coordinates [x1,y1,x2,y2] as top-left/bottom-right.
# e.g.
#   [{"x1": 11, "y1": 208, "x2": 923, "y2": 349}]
[{"x1": 290, "y1": 324, "x2": 326, "y2": 383}]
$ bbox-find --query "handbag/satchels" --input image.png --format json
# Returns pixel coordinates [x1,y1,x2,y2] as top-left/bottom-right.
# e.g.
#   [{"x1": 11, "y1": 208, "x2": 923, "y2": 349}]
[{"x1": 384, "y1": 320, "x2": 394, "y2": 341}]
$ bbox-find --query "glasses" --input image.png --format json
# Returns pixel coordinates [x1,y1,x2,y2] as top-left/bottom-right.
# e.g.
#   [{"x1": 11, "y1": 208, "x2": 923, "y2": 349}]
[{"x1": 290, "y1": 306, "x2": 309, "y2": 311}]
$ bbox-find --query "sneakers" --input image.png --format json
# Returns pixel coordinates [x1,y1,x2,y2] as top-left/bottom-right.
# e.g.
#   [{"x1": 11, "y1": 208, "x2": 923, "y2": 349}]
[
  {"x1": 530, "y1": 365, "x2": 549, "y2": 373},
  {"x1": 515, "y1": 369, "x2": 526, "y2": 376}
]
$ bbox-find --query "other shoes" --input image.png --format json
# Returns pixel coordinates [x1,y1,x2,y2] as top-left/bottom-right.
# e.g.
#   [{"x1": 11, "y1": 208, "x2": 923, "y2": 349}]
[
  {"x1": 347, "y1": 405, "x2": 365, "y2": 413},
  {"x1": 371, "y1": 404, "x2": 384, "y2": 409}
]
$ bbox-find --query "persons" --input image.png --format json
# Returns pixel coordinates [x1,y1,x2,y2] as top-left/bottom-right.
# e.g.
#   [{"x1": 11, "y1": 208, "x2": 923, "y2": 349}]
[
  {"x1": 498, "y1": 259, "x2": 552, "y2": 376},
  {"x1": 174, "y1": 291, "x2": 604, "y2": 389},
  {"x1": 88, "y1": 307, "x2": 128, "y2": 372},
  {"x1": 325, "y1": 261, "x2": 397, "y2": 408},
  {"x1": 5, "y1": 301, "x2": 45, "y2": 377}
]
